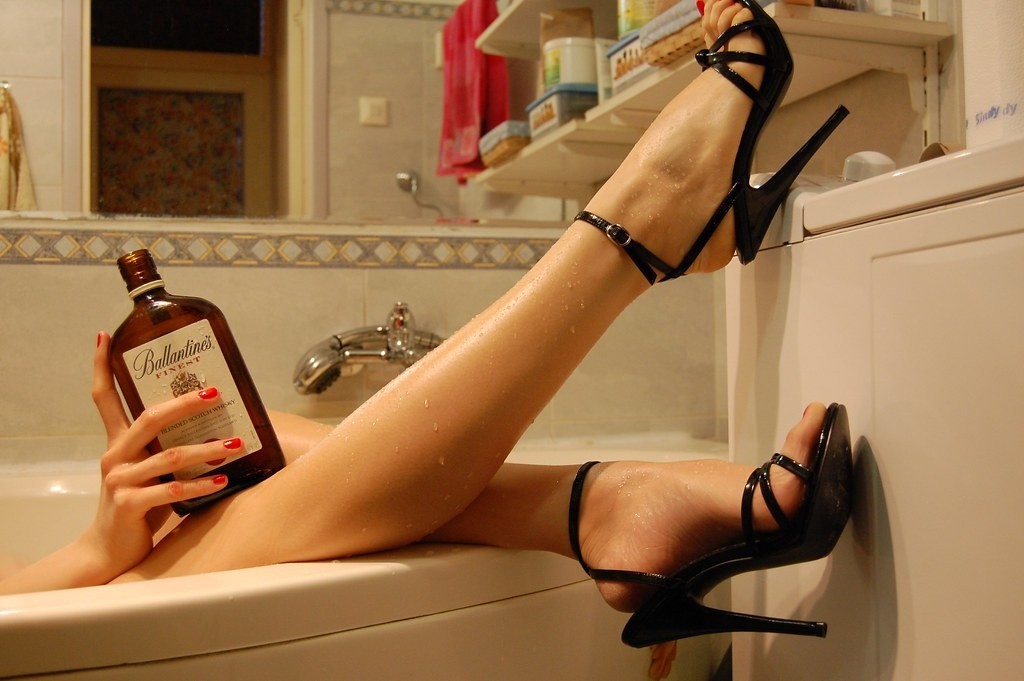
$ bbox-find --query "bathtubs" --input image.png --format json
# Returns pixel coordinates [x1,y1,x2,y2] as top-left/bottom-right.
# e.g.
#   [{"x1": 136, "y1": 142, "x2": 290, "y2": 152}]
[{"x1": 0, "y1": 429, "x2": 732, "y2": 678}]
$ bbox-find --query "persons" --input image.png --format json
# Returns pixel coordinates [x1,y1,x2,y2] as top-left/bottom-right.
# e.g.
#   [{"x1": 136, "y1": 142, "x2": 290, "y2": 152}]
[{"x1": 0, "y1": 0, "x2": 855, "y2": 648}]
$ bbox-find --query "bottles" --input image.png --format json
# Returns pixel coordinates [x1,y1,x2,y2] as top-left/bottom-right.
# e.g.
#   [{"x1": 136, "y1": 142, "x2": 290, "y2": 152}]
[{"x1": 108, "y1": 248, "x2": 284, "y2": 517}]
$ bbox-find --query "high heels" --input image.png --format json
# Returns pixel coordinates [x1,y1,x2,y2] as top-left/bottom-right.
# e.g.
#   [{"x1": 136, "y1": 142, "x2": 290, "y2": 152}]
[
  {"x1": 574, "y1": 0, "x2": 851, "y2": 285},
  {"x1": 568, "y1": 403, "x2": 854, "y2": 649}
]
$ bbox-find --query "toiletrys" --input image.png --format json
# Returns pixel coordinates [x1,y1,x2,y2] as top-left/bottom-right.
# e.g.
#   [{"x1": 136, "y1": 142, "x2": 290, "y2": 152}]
[
  {"x1": 543, "y1": 37, "x2": 592, "y2": 88},
  {"x1": 840, "y1": 150, "x2": 895, "y2": 185},
  {"x1": 595, "y1": 35, "x2": 621, "y2": 102}
]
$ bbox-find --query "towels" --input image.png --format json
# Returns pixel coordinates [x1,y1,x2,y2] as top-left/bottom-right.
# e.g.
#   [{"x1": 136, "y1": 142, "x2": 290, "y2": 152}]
[
  {"x1": 1, "y1": 88, "x2": 38, "y2": 212},
  {"x1": 437, "y1": 0, "x2": 509, "y2": 175}
]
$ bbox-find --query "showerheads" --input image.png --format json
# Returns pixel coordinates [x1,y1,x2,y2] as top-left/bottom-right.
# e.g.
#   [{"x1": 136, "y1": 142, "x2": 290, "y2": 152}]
[
  {"x1": 290, "y1": 328, "x2": 447, "y2": 394},
  {"x1": 397, "y1": 171, "x2": 419, "y2": 192}
]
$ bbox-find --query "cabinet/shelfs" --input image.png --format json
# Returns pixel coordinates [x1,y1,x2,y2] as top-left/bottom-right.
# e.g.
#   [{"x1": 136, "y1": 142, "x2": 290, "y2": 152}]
[
  {"x1": 475, "y1": 0, "x2": 949, "y2": 199},
  {"x1": 723, "y1": 137, "x2": 1024, "y2": 681}
]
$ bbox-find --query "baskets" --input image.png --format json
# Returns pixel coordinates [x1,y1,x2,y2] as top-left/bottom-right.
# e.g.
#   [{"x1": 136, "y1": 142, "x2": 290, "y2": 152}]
[{"x1": 641, "y1": 0, "x2": 707, "y2": 68}]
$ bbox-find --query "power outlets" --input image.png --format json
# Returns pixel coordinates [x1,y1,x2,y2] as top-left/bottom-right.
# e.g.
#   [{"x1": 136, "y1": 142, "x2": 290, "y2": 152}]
[{"x1": 358, "y1": 96, "x2": 389, "y2": 125}]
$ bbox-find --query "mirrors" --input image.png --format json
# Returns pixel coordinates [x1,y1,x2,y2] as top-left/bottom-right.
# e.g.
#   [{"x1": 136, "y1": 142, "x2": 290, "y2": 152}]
[{"x1": 0, "y1": 0, "x2": 588, "y2": 221}]
